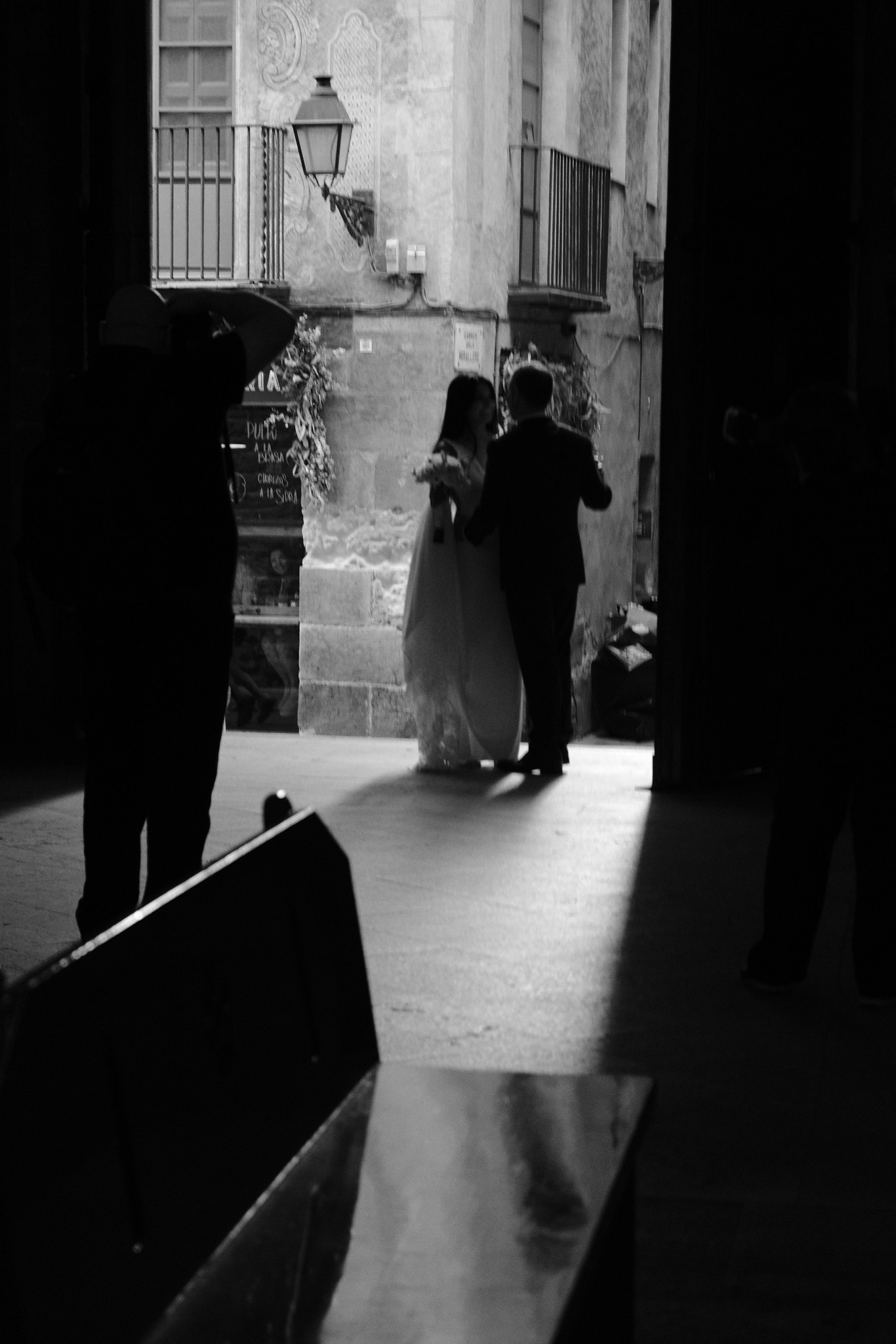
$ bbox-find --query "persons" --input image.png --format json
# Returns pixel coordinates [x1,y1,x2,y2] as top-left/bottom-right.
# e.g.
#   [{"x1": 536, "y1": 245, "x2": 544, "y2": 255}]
[
  {"x1": 466, "y1": 367, "x2": 612, "y2": 778},
  {"x1": 250, "y1": 546, "x2": 299, "y2": 721},
  {"x1": 35, "y1": 288, "x2": 298, "y2": 945},
  {"x1": 405, "y1": 373, "x2": 525, "y2": 775}
]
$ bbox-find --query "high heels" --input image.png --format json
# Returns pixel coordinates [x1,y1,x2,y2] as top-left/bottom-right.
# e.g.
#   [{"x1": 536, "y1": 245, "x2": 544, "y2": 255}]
[{"x1": 509, "y1": 747, "x2": 570, "y2": 777}]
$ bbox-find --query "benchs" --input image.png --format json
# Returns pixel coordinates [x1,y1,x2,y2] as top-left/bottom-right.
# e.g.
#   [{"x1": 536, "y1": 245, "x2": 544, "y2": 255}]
[{"x1": 0, "y1": 791, "x2": 657, "y2": 1344}]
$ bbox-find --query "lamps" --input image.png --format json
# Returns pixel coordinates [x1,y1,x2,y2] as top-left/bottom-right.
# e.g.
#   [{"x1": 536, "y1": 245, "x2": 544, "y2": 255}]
[{"x1": 283, "y1": 72, "x2": 375, "y2": 248}]
